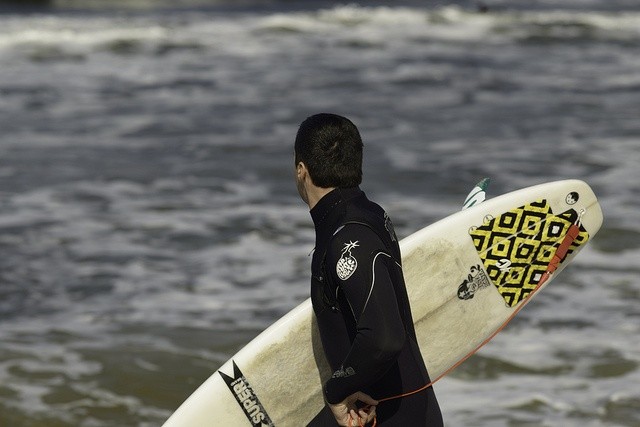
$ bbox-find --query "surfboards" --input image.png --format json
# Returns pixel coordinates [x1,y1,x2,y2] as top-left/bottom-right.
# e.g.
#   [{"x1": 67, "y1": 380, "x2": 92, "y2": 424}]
[{"x1": 161, "y1": 178, "x2": 603, "y2": 427}]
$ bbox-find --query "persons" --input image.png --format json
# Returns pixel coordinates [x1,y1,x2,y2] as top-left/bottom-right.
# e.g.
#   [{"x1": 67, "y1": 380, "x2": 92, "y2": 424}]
[{"x1": 295, "y1": 113, "x2": 443, "y2": 427}]
